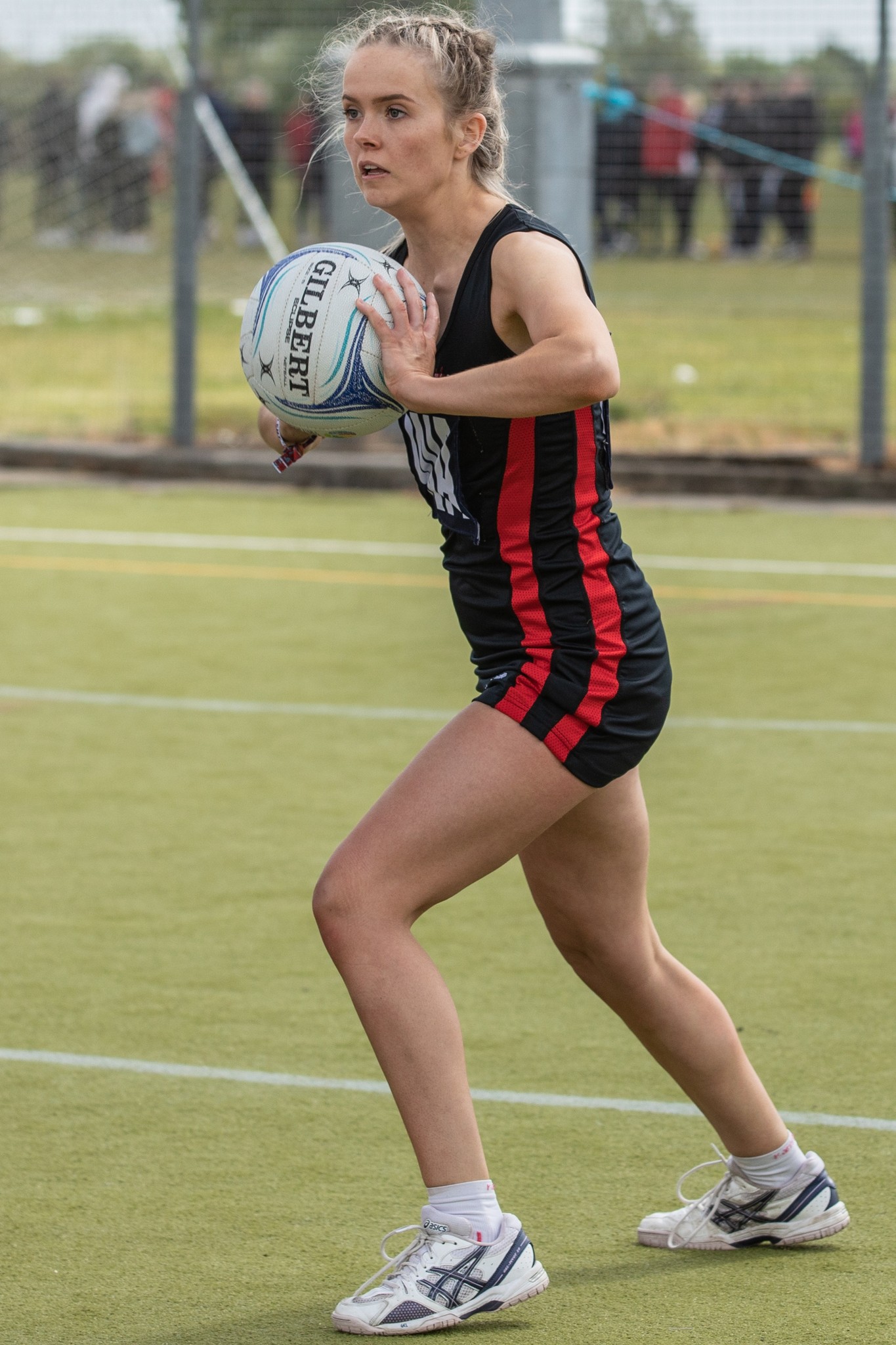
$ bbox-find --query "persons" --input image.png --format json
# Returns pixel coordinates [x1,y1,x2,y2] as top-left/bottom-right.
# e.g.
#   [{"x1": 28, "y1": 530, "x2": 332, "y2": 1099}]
[
  {"x1": 34, "y1": 62, "x2": 866, "y2": 264},
  {"x1": 255, "y1": 0, "x2": 852, "y2": 1336}
]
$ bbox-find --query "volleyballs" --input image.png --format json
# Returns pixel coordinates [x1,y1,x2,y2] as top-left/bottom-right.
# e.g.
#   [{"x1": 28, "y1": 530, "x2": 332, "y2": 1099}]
[{"x1": 239, "y1": 241, "x2": 427, "y2": 439}]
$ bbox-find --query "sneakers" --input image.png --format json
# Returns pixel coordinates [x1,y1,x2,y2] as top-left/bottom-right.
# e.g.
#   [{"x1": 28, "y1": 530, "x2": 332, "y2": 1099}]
[
  {"x1": 637, "y1": 1133, "x2": 851, "y2": 1249},
  {"x1": 330, "y1": 1205, "x2": 549, "y2": 1334}
]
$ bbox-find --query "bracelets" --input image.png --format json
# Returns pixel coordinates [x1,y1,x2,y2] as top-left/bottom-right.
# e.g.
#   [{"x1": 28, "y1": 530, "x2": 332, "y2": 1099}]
[{"x1": 272, "y1": 417, "x2": 317, "y2": 473}]
